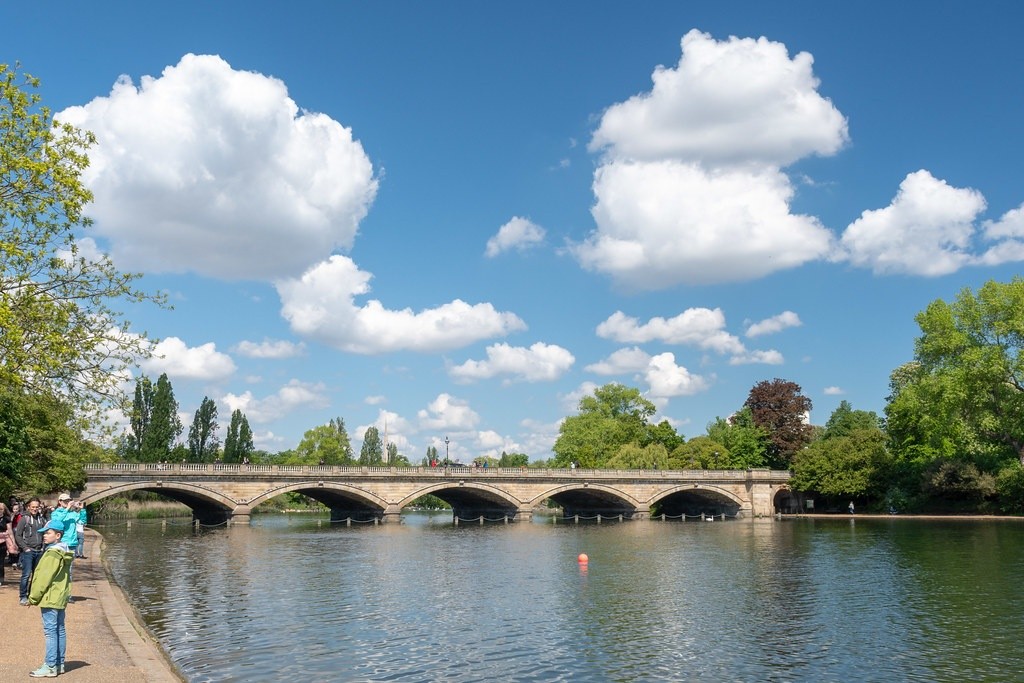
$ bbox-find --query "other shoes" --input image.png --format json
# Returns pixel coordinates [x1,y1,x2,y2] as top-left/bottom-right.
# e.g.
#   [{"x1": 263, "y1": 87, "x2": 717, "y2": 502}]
[
  {"x1": 68, "y1": 597, "x2": 75, "y2": 604},
  {"x1": 20, "y1": 596, "x2": 28, "y2": 606},
  {"x1": 57, "y1": 664, "x2": 65, "y2": 674},
  {"x1": 76, "y1": 555, "x2": 81, "y2": 558},
  {"x1": 29, "y1": 663, "x2": 58, "y2": 677},
  {"x1": 80, "y1": 555, "x2": 87, "y2": 559}
]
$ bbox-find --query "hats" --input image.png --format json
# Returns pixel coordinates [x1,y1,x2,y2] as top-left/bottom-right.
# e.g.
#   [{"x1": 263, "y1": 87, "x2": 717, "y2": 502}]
[
  {"x1": 37, "y1": 519, "x2": 64, "y2": 533},
  {"x1": 57, "y1": 494, "x2": 71, "y2": 501}
]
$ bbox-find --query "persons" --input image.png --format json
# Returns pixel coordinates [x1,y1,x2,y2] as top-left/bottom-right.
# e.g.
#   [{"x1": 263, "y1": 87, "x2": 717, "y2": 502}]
[
  {"x1": 473, "y1": 460, "x2": 489, "y2": 474},
  {"x1": 242, "y1": 457, "x2": 249, "y2": 468},
  {"x1": 319, "y1": 458, "x2": 324, "y2": 470},
  {"x1": 571, "y1": 459, "x2": 579, "y2": 474},
  {"x1": 25, "y1": 520, "x2": 74, "y2": 678},
  {"x1": 215, "y1": 458, "x2": 223, "y2": 471},
  {"x1": 431, "y1": 458, "x2": 447, "y2": 472},
  {"x1": 0, "y1": 493, "x2": 88, "y2": 606},
  {"x1": 849, "y1": 501, "x2": 854, "y2": 515}
]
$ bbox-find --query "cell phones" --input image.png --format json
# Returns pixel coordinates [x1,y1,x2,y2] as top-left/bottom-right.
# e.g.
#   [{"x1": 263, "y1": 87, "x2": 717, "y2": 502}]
[{"x1": 73, "y1": 502, "x2": 79, "y2": 508}]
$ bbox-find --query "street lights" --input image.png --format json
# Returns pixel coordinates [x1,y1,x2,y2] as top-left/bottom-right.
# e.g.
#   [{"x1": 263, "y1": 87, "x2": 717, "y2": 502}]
[
  {"x1": 444, "y1": 436, "x2": 450, "y2": 468},
  {"x1": 386, "y1": 444, "x2": 390, "y2": 468}
]
